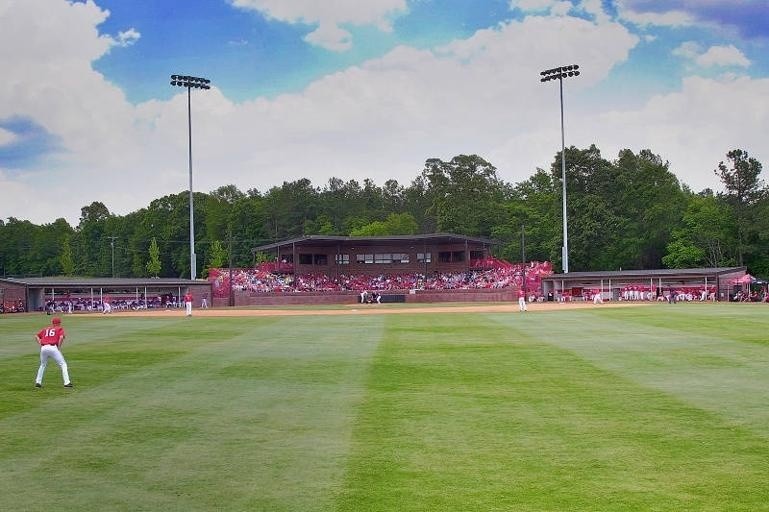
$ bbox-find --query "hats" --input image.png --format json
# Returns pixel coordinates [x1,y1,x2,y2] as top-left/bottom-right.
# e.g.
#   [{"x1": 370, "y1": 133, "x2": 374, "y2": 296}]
[{"x1": 52, "y1": 317, "x2": 62, "y2": 324}]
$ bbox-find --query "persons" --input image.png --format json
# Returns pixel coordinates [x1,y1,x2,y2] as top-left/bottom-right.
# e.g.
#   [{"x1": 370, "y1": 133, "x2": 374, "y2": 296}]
[
  {"x1": 371, "y1": 291, "x2": 382, "y2": 303},
  {"x1": 200, "y1": 292, "x2": 209, "y2": 309},
  {"x1": 43, "y1": 293, "x2": 186, "y2": 315},
  {"x1": 527, "y1": 283, "x2": 769, "y2": 304},
  {"x1": 33, "y1": 317, "x2": 74, "y2": 388},
  {"x1": 183, "y1": 291, "x2": 194, "y2": 317},
  {"x1": 516, "y1": 287, "x2": 528, "y2": 313},
  {"x1": 360, "y1": 290, "x2": 367, "y2": 303},
  {"x1": 219, "y1": 261, "x2": 552, "y2": 293}
]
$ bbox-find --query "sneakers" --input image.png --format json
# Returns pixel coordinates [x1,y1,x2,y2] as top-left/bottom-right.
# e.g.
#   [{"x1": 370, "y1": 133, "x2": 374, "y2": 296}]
[{"x1": 35, "y1": 382, "x2": 74, "y2": 388}]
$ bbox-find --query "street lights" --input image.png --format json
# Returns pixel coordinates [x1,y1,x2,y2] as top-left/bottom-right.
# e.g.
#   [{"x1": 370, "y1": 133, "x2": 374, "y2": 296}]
[
  {"x1": 538, "y1": 62, "x2": 579, "y2": 273},
  {"x1": 170, "y1": 74, "x2": 212, "y2": 280}
]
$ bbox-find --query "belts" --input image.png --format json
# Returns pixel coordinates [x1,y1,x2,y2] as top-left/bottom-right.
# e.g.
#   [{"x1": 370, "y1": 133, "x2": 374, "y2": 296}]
[{"x1": 41, "y1": 343, "x2": 55, "y2": 346}]
